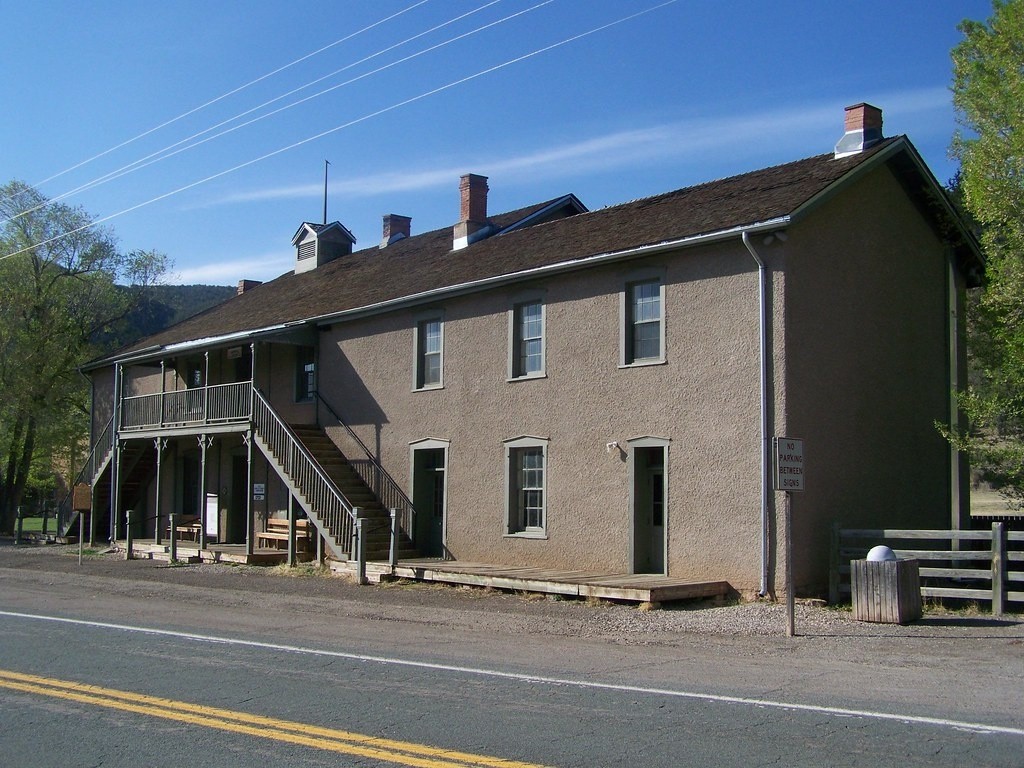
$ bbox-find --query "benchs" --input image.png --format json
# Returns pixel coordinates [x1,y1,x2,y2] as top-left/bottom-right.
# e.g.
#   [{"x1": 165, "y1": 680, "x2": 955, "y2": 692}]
[
  {"x1": 165, "y1": 515, "x2": 201, "y2": 543},
  {"x1": 257, "y1": 518, "x2": 310, "y2": 553}
]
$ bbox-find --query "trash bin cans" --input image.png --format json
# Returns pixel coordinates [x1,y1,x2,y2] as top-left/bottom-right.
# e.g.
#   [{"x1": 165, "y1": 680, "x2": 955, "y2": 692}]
[{"x1": 849, "y1": 545, "x2": 920, "y2": 624}]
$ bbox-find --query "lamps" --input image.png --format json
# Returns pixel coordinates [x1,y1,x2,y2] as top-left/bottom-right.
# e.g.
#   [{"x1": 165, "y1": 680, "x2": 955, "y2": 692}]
[{"x1": 606, "y1": 442, "x2": 619, "y2": 453}]
